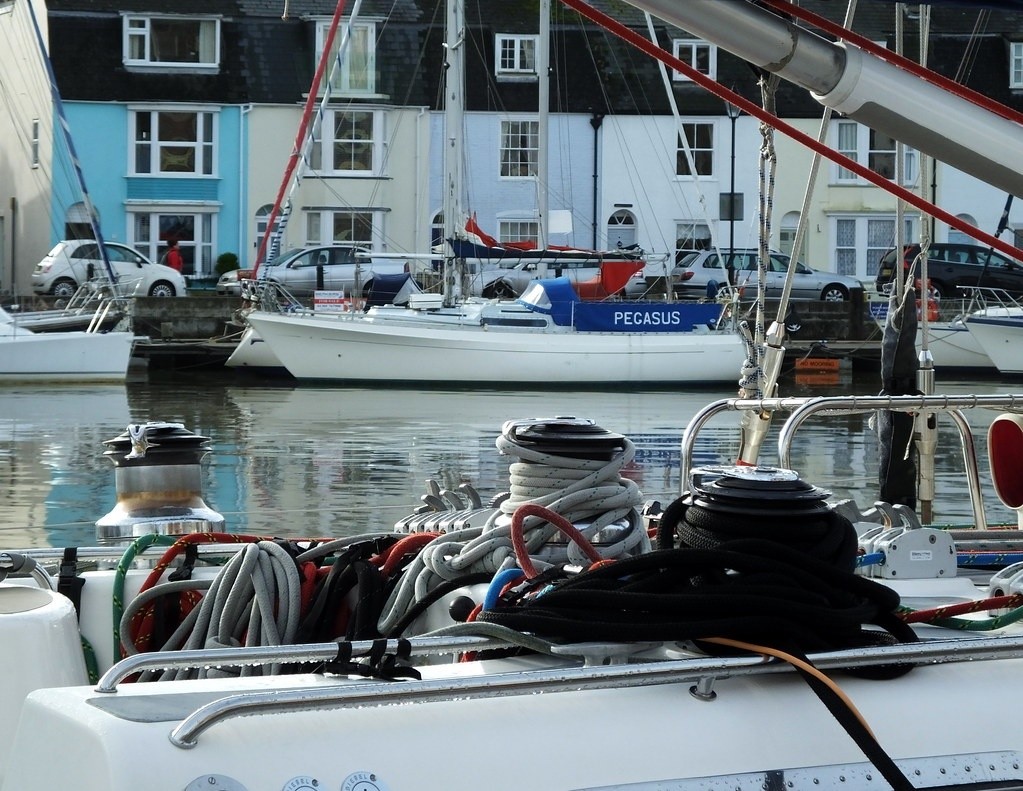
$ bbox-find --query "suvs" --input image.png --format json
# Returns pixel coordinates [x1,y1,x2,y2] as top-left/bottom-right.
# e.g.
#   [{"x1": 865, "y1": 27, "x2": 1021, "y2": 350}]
[
  {"x1": 876, "y1": 240, "x2": 1023, "y2": 303},
  {"x1": 666, "y1": 247, "x2": 872, "y2": 303}
]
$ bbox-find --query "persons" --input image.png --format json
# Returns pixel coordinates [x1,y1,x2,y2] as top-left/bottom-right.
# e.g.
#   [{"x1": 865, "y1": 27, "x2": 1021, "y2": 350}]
[{"x1": 167, "y1": 238, "x2": 182, "y2": 270}]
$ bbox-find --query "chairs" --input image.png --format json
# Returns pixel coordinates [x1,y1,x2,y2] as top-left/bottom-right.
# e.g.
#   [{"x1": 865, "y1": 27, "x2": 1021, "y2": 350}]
[
  {"x1": 319, "y1": 255, "x2": 327, "y2": 265},
  {"x1": 954, "y1": 254, "x2": 961, "y2": 262},
  {"x1": 770, "y1": 259, "x2": 776, "y2": 271},
  {"x1": 746, "y1": 262, "x2": 758, "y2": 270}
]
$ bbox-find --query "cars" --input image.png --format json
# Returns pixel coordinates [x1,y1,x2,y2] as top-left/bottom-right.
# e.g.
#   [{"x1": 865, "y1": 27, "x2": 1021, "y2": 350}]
[
  {"x1": 30, "y1": 239, "x2": 188, "y2": 298},
  {"x1": 216, "y1": 242, "x2": 409, "y2": 309}
]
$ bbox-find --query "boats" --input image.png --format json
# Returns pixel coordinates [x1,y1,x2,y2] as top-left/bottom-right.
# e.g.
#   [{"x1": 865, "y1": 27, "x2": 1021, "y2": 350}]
[{"x1": 1, "y1": 0, "x2": 1023, "y2": 790}]
